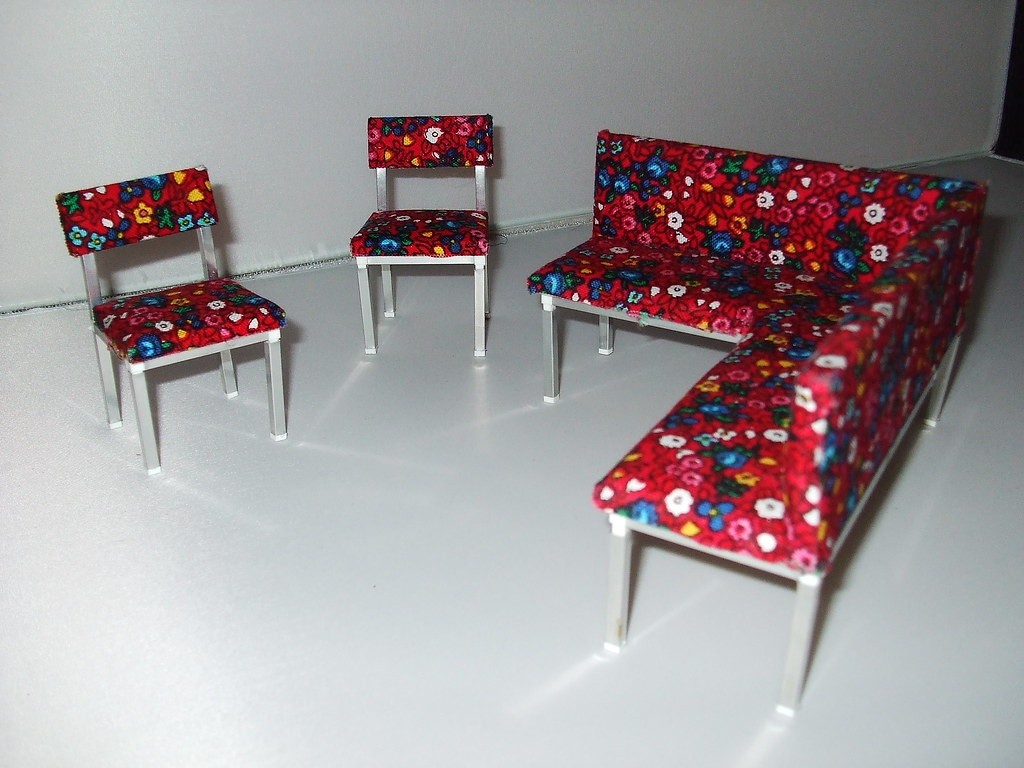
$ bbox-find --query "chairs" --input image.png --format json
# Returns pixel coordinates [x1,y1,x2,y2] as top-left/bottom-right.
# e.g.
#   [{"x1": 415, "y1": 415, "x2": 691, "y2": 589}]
[
  {"x1": 349, "y1": 114, "x2": 495, "y2": 355},
  {"x1": 55, "y1": 164, "x2": 290, "y2": 476}
]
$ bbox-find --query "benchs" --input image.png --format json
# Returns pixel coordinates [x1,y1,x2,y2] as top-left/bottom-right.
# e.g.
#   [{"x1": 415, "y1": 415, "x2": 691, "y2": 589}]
[{"x1": 532, "y1": 130, "x2": 987, "y2": 714}]
